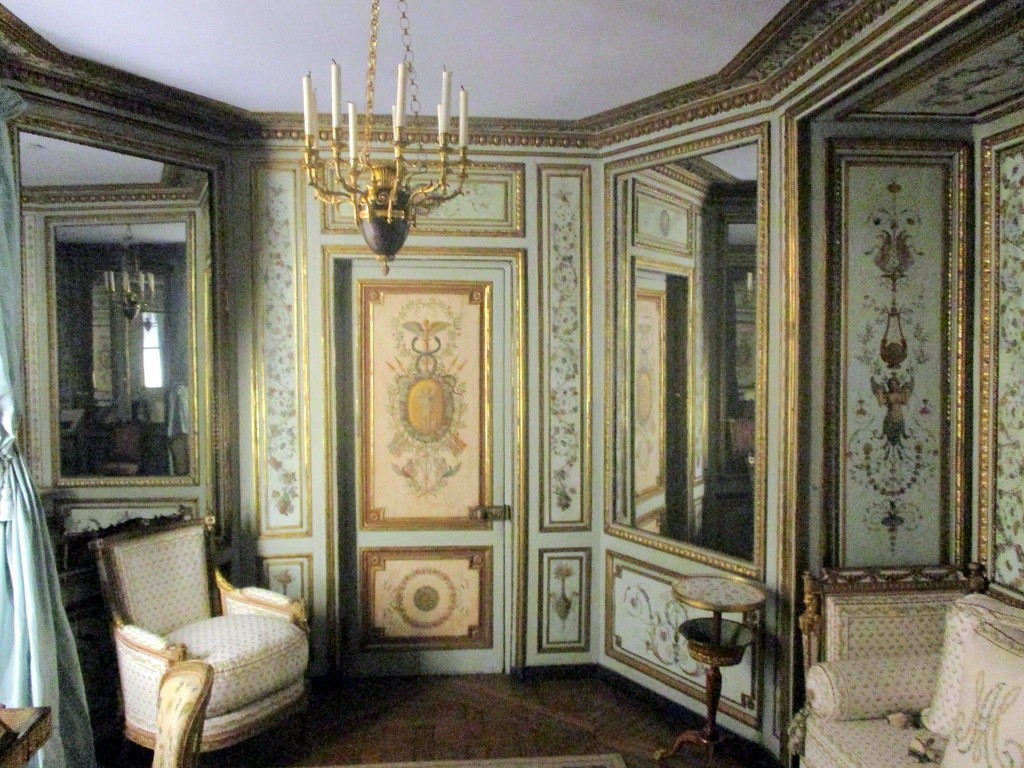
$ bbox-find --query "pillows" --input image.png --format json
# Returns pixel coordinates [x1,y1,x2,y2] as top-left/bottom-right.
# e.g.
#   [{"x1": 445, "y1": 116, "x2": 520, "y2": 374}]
[
  {"x1": 920, "y1": 593, "x2": 1023, "y2": 741},
  {"x1": 941, "y1": 624, "x2": 1024, "y2": 768},
  {"x1": 805, "y1": 653, "x2": 939, "y2": 722}
]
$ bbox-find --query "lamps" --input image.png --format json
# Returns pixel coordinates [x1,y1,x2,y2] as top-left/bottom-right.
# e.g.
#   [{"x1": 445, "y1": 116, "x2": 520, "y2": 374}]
[
  {"x1": 102, "y1": 225, "x2": 155, "y2": 322},
  {"x1": 303, "y1": 0, "x2": 469, "y2": 274}
]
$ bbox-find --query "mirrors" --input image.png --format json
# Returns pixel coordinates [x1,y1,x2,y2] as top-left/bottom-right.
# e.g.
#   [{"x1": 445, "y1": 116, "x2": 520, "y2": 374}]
[
  {"x1": 45, "y1": 214, "x2": 201, "y2": 489},
  {"x1": 602, "y1": 118, "x2": 772, "y2": 584}
]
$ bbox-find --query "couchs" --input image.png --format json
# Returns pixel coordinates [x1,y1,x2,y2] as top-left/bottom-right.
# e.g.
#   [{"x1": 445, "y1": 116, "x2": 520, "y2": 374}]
[
  {"x1": 785, "y1": 562, "x2": 1024, "y2": 768},
  {"x1": 86, "y1": 513, "x2": 313, "y2": 767}
]
